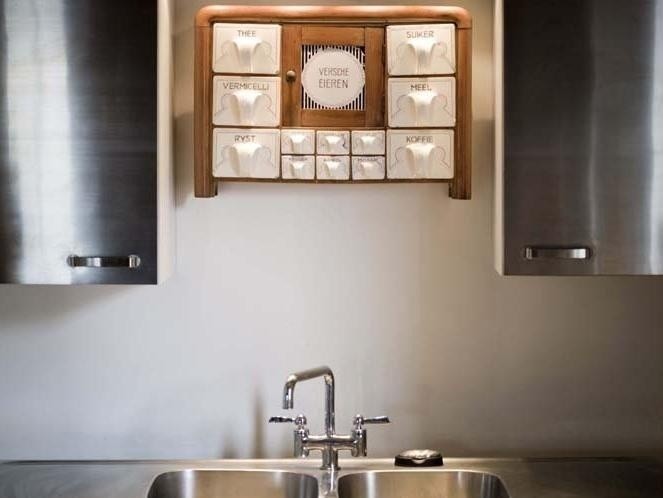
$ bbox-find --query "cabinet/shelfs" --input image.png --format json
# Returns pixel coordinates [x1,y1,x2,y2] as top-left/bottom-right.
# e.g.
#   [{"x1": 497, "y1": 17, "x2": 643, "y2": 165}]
[
  {"x1": 493, "y1": 0, "x2": 663, "y2": 276},
  {"x1": 0, "y1": 0, "x2": 175, "y2": 285}
]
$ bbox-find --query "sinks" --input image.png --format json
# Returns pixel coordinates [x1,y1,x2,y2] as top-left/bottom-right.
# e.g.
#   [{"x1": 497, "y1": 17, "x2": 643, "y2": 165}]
[
  {"x1": 145, "y1": 466, "x2": 319, "y2": 498},
  {"x1": 336, "y1": 464, "x2": 510, "y2": 497}
]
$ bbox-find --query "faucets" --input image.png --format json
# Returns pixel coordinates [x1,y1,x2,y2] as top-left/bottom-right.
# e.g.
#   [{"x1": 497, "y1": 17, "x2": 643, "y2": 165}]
[{"x1": 281, "y1": 364, "x2": 339, "y2": 473}]
